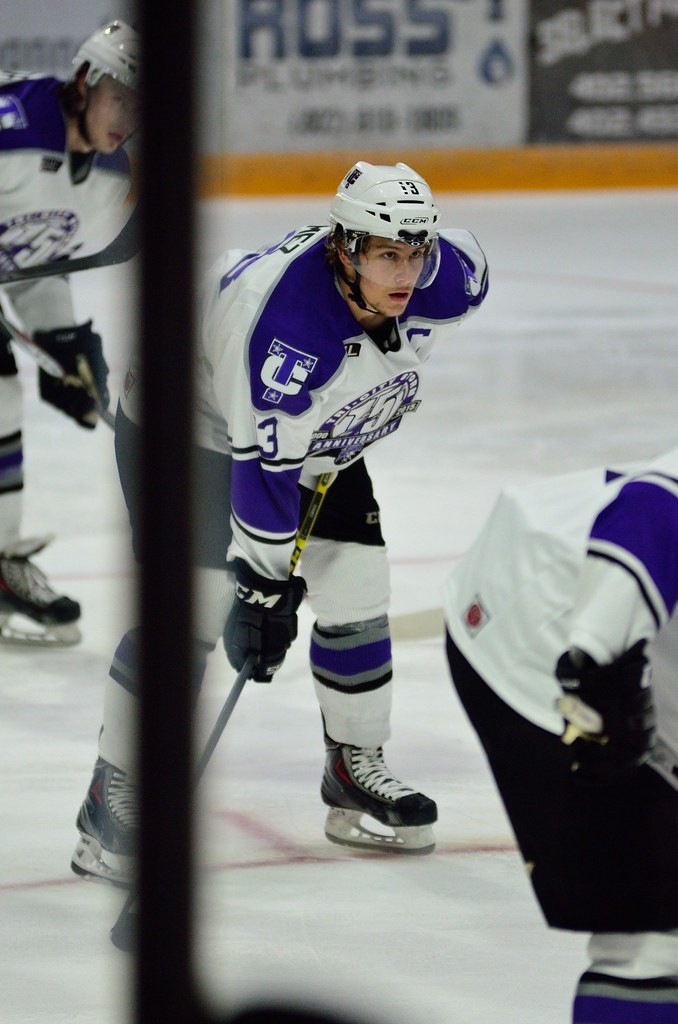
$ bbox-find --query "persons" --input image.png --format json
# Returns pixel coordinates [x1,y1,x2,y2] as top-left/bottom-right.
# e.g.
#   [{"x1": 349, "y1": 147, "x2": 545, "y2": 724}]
[
  {"x1": 447, "y1": 453, "x2": 678, "y2": 1024},
  {"x1": 1, "y1": 21, "x2": 134, "y2": 645},
  {"x1": 73, "y1": 161, "x2": 489, "y2": 892}
]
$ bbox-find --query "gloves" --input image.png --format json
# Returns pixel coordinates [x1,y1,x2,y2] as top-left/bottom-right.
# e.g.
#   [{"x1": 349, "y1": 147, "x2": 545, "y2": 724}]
[
  {"x1": 221, "y1": 557, "x2": 308, "y2": 683},
  {"x1": 33, "y1": 317, "x2": 112, "y2": 430},
  {"x1": 549, "y1": 639, "x2": 654, "y2": 805}
]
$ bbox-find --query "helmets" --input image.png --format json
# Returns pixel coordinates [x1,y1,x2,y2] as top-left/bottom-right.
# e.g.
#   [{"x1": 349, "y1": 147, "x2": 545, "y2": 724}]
[
  {"x1": 324, "y1": 161, "x2": 440, "y2": 255},
  {"x1": 69, "y1": 19, "x2": 143, "y2": 90}
]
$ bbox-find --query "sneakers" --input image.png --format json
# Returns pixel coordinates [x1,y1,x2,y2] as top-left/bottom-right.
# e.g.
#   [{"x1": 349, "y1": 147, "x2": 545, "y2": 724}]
[
  {"x1": 0, "y1": 529, "x2": 83, "y2": 646},
  {"x1": 70, "y1": 755, "x2": 141, "y2": 890},
  {"x1": 320, "y1": 734, "x2": 439, "y2": 855}
]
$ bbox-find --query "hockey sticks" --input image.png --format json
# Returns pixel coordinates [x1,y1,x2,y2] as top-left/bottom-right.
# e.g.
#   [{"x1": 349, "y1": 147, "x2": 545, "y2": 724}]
[
  {"x1": 110, "y1": 470, "x2": 341, "y2": 955},
  {"x1": 0, "y1": 308, "x2": 116, "y2": 434},
  {"x1": 0, "y1": 193, "x2": 142, "y2": 288}
]
